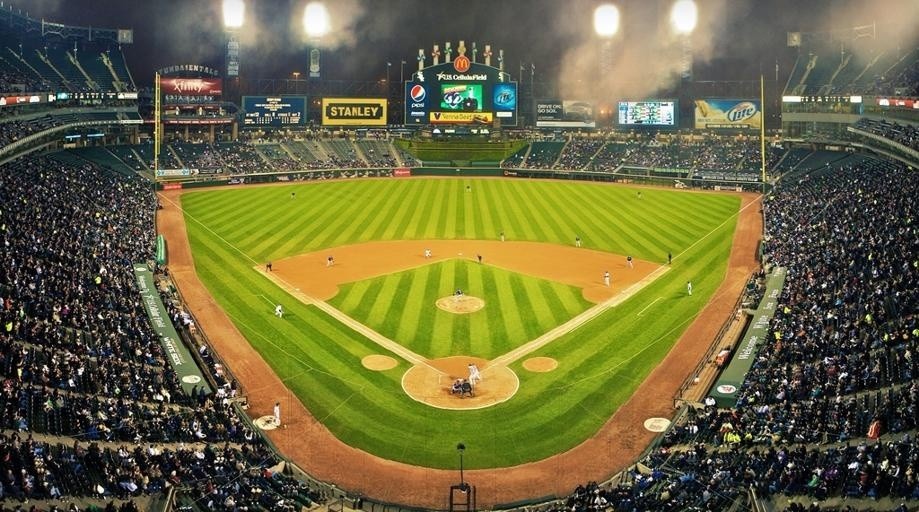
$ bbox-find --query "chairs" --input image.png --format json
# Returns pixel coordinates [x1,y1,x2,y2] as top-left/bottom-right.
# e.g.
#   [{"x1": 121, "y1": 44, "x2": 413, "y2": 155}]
[
  {"x1": 526, "y1": 50, "x2": 918, "y2": 512},
  {"x1": 2, "y1": 17, "x2": 330, "y2": 510},
  {"x1": 311, "y1": 123, "x2": 882, "y2": 189}
]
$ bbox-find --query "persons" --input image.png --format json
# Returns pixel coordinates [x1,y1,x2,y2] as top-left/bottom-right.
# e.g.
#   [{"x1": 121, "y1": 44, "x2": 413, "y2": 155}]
[
  {"x1": 575, "y1": 234, "x2": 671, "y2": 287},
  {"x1": 453, "y1": 363, "x2": 483, "y2": 397},
  {"x1": 503, "y1": 129, "x2": 772, "y2": 193},
  {"x1": 805, "y1": 71, "x2": 919, "y2": 160},
  {"x1": 566, "y1": 431, "x2": 919, "y2": 512},
  {"x1": 2, "y1": 70, "x2": 333, "y2": 511},
  {"x1": 426, "y1": 233, "x2": 505, "y2": 300},
  {"x1": 687, "y1": 280, "x2": 693, "y2": 297},
  {"x1": 659, "y1": 161, "x2": 918, "y2": 448},
  {"x1": 158, "y1": 101, "x2": 415, "y2": 182}
]
